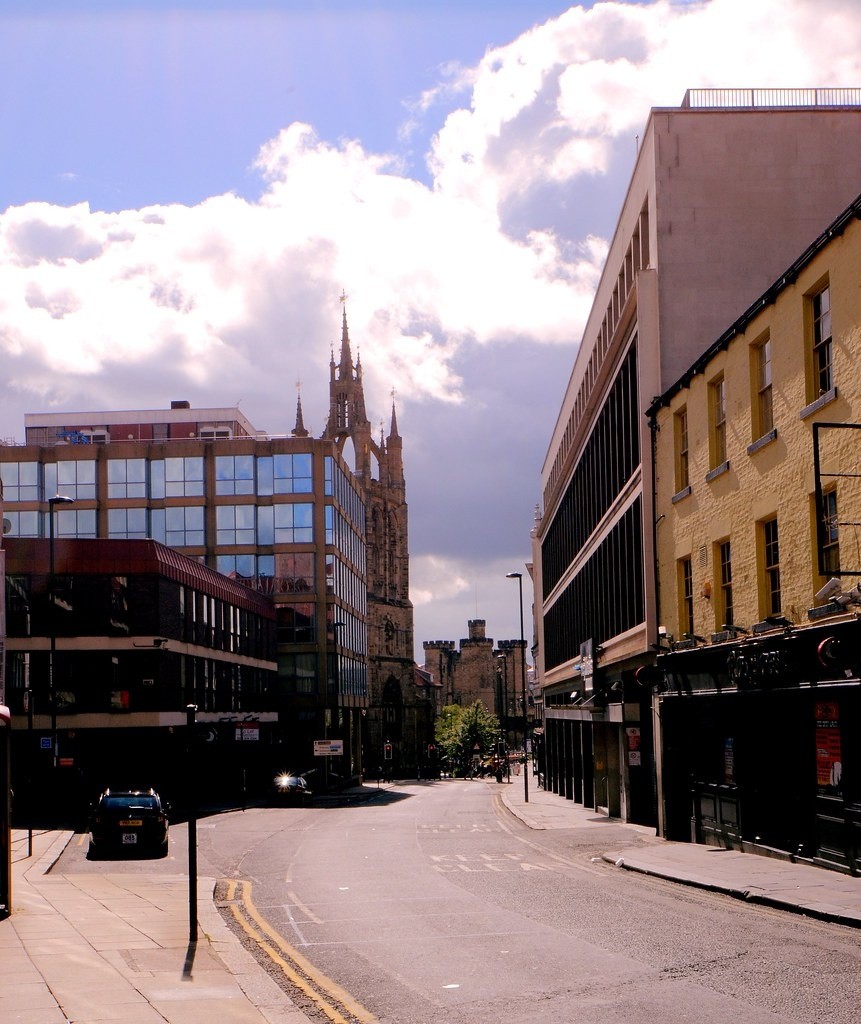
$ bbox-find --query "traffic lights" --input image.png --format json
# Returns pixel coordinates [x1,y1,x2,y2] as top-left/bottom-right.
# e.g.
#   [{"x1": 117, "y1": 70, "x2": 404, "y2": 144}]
[
  {"x1": 384, "y1": 744, "x2": 393, "y2": 760},
  {"x1": 427, "y1": 744, "x2": 434, "y2": 758}
]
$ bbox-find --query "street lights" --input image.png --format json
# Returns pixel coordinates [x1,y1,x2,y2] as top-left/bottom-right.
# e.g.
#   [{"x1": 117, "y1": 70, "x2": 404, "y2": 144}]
[
  {"x1": 330, "y1": 620, "x2": 345, "y2": 710},
  {"x1": 506, "y1": 573, "x2": 530, "y2": 803},
  {"x1": 42, "y1": 495, "x2": 76, "y2": 771}
]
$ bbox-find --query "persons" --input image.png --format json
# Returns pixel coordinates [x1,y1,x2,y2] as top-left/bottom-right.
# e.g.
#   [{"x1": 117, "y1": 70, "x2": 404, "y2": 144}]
[{"x1": 477, "y1": 760, "x2": 507, "y2": 783}]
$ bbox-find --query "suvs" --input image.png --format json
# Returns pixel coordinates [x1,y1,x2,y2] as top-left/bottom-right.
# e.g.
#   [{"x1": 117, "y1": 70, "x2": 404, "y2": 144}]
[{"x1": 88, "y1": 788, "x2": 170, "y2": 856}]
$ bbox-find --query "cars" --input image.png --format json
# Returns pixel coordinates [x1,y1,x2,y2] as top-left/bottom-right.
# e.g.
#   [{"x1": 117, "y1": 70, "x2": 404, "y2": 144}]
[{"x1": 274, "y1": 776, "x2": 315, "y2": 807}]
